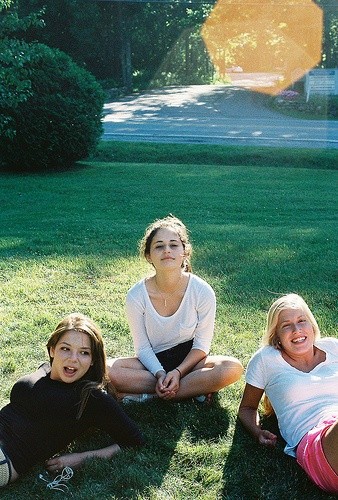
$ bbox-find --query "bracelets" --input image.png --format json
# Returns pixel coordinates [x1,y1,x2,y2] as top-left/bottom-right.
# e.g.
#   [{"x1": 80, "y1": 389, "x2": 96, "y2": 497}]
[{"x1": 175, "y1": 368, "x2": 182, "y2": 380}]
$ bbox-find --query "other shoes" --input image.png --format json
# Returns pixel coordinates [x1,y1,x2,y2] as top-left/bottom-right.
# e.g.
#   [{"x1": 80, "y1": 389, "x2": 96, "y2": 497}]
[
  {"x1": 195, "y1": 393, "x2": 213, "y2": 406},
  {"x1": 123, "y1": 394, "x2": 147, "y2": 404}
]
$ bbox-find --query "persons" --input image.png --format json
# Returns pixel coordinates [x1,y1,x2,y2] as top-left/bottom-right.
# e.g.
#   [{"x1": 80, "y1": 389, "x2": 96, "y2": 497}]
[
  {"x1": 0, "y1": 312, "x2": 142, "y2": 487},
  {"x1": 238, "y1": 294, "x2": 338, "y2": 494},
  {"x1": 107, "y1": 215, "x2": 244, "y2": 403}
]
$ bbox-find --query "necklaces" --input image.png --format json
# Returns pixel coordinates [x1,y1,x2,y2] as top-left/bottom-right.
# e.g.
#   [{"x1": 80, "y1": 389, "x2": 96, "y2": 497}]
[{"x1": 153, "y1": 274, "x2": 185, "y2": 307}]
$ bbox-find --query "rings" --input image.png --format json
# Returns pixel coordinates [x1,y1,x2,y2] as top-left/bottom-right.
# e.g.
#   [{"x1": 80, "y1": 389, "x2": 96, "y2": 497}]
[{"x1": 264, "y1": 440, "x2": 267, "y2": 444}]
